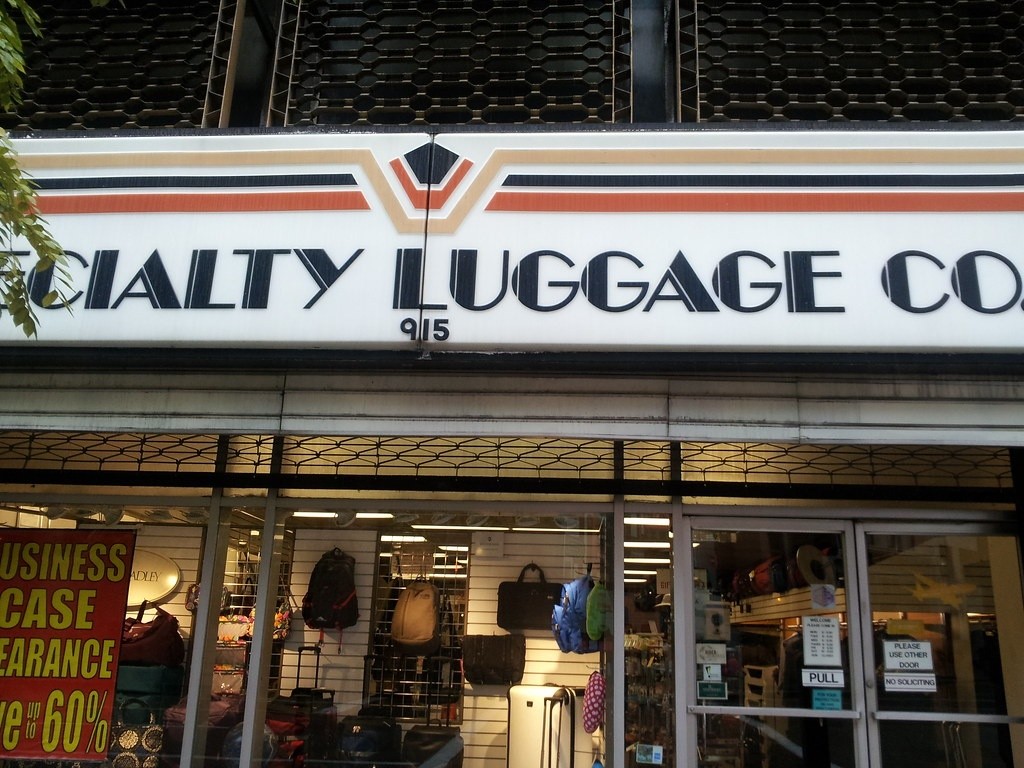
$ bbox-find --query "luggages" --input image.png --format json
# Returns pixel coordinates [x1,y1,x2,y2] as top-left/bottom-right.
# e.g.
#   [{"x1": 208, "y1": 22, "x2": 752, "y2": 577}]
[{"x1": 265, "y1": 646, "x2": 339, "y2": 768}]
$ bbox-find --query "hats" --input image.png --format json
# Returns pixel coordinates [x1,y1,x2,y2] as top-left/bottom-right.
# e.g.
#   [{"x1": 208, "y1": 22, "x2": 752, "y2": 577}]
[{"x1": 655, "y1": 594, "x2": 672, "y2": 607}]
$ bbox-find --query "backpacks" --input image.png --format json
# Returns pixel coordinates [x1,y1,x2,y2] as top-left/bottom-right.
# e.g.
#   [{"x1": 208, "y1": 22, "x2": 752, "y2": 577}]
[
  {"x1": 552, "y1": 563, "x2": 599, "y2": 655},
  {"x1": 302, "y1": 548, "x2": 359, "y2": 630}
]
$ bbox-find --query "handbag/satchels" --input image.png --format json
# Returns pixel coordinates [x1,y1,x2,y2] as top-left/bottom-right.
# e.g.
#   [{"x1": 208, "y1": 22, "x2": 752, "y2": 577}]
[
  {"x1": 464, "y1": 634, "x2": 526, "y2": 685},
  {"x1": 118, "y1": 600, "x2": 185, "y2": 667},
  {"x1": 109, "y1": 698, "x2": 164, "y2": 768},
  {"x1": 331, "y1": 715, "x2": 402, "y2": 768},
  {"x1": 162, "y1": 693, "x2": 237, "y2": 758},
  {"x1": 186, "y1": 575, "x2": 292, "y2": 700},
  {"x1": 401, "y1": 719, "x2": 464, "y2": 768},
  {"x1": 370, "y1": 576, "x2": 463, "y2": 726},
  {"x1": 497, "y1": 563, "x2": 562, "y2": 630}
]
